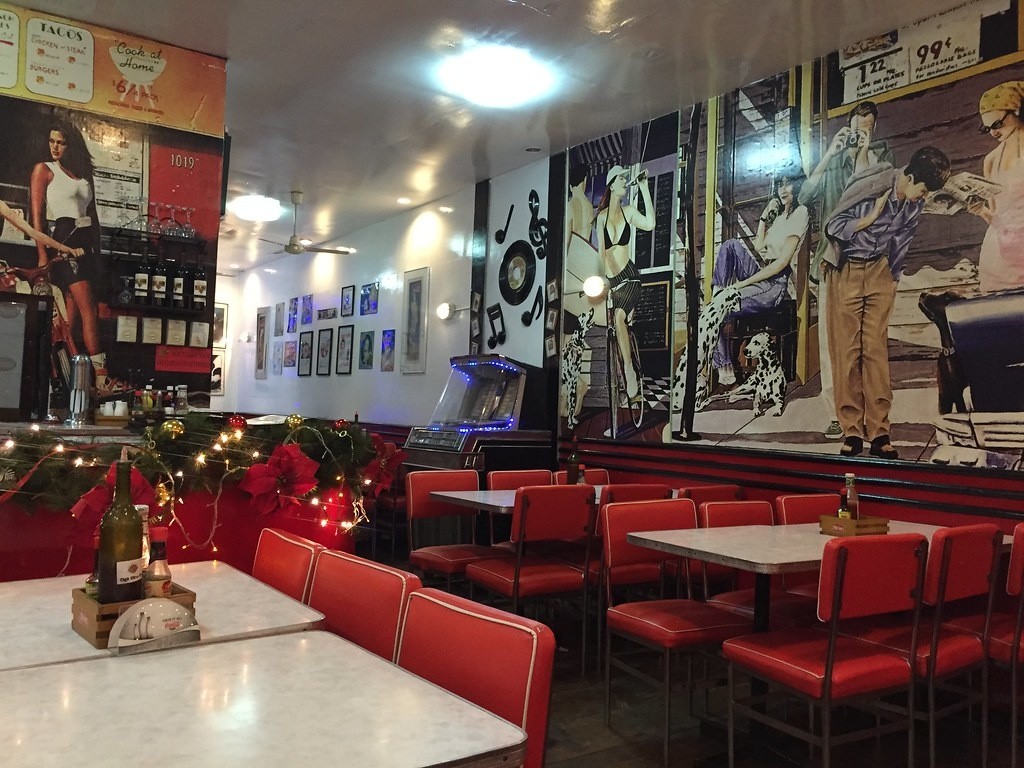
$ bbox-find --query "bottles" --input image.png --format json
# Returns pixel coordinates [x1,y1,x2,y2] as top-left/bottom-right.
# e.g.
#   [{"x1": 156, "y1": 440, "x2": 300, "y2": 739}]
[
  {"x1": 145, "y1": 527, "x2": 172, "y2": 599},
  {"x1": 97, "y1": 461, "x2": 145, "y2": 603},
  {"x1": 624, "y1": 169, "x2": 649, "y2": 189},
  {"x1": 576, "y1": 464, "x2": 587, "y2": 485},
  {"x1": 85, "y1": 536, "x2": 98, "y2": 599},
  {"x1": 566, "y1": 436, "x2": 582, "y2": 485},
  {"x1": 97, "y1": 384, "x2": 190, "y2": 419},
  {"x1": 845, "y1": 473, "x2": 859, "y2": 521},
  {"x1": 133, "y1": 245, "x2": 207, "y2": 310},
  {"x1": 350, "y1": 414, "x2": 362, "y2": 429},
  {"x1": 837, "y1": 489, "x2": 852, "y2": 520},
  {"x1": 134, "y1": 505, "x2": 150, "y2": 572}
]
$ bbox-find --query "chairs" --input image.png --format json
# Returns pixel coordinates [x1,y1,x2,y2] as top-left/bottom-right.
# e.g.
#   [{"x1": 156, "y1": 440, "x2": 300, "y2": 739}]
[{"x1": 252, "y1": 464, "x2": 1024, "y2": 768}]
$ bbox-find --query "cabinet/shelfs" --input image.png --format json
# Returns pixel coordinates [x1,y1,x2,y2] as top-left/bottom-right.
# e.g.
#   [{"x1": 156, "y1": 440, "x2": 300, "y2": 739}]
[{"x1": 100, "y1": 225, "x2": 207, "y2": 315}]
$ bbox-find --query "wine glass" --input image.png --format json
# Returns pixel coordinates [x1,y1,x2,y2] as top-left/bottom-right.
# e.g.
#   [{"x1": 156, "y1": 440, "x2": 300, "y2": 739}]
[{"x1": 114, "y1": 196, "x2": 195, "y2": 239}]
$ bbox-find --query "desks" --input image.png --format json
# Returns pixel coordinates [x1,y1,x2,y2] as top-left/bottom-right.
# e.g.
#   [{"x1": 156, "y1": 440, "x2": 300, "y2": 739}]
[
  {"x1": 423, "y1": 484, "x2": 678, "y2": 655},
  {"x1": 0, "y1": 615, "x2": 527, "y2": 768},
  {"x1": 93, "y1": 408, "x2": 132, "y2": 427},
  {"x1": 0, "y1": 559, "x2": 325, "y2": 671},
  {"x1": 627, "y1": 518, "x2": 1014, "y2": 766}
]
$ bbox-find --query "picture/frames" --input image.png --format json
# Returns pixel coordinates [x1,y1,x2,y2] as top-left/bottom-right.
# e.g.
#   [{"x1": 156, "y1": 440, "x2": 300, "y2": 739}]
[
  {"x1": 211, "y1": 349, "x2": 224, "y2": 396},
  {"x1": 317, "y1": 327, "x2": 333, "y2": 375},
  {"x1": 297, "y1": 330, "x2": 313, "y2": 376},
  {"x1": 340, "y1": 285, "x2": 355, "y2": 316},
  {"x1": 336, "y1": 325, "x2": 354, "y2": 374},
  {"x1": 214, "y1": 301, "x2": 229, "y2": 348},
  {"x1": 399, "y1": 266, "x2": 430, "y2": 374}
]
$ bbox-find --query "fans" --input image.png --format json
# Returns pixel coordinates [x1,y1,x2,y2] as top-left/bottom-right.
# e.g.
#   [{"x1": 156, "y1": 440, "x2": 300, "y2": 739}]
[{"x1": 257, "y1": 190, "x2": 350, "y2": 256}]
